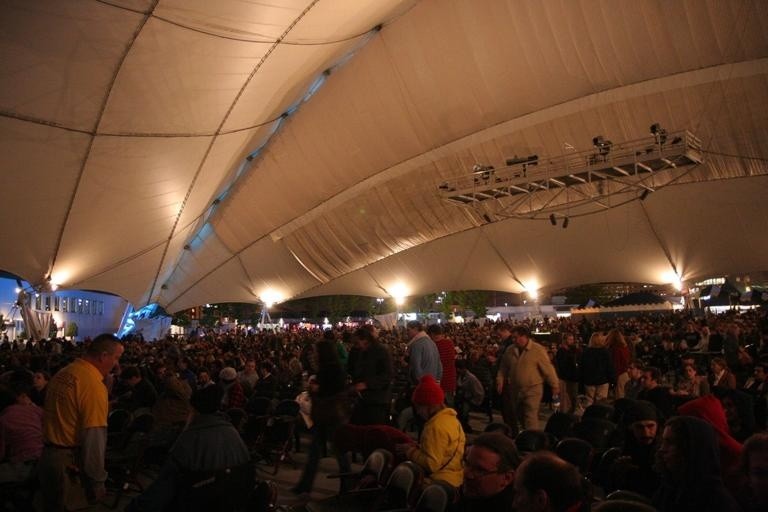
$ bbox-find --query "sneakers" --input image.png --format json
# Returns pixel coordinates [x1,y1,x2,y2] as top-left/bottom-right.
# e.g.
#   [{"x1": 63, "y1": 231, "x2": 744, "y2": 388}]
[{"x1": 292, "y1": 487, "x2": 312, "y2": 494}]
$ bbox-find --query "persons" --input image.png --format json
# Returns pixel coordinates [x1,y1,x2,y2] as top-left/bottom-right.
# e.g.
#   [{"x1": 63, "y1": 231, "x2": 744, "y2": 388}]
[{"x1": 0, "y1": 300, "x2": 768, "y2": 512}]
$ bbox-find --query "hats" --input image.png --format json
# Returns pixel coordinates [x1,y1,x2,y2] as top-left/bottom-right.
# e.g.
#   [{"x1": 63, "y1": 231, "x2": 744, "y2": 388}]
[
  {"x1": 412, "y1": 375, "x2": 445, "y2": 405},
  {"x1": 219, "y1": 367, "x2": 237, "y2": 381},
  {"x1": 332, "y1": 424, "x2": 355, "y2": 455},
  {"x1": 189, "y1": 383, "x2": 224, "y2": 415}
]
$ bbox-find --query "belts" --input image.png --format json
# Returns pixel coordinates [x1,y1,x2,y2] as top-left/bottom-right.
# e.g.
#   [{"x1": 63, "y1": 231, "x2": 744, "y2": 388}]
[{"x1": 25, "y1": 459, "x2": 38, "y2": 466}]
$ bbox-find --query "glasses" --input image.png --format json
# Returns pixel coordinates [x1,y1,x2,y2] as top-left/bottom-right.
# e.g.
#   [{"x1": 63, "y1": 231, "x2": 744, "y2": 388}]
[{"x1": 460, "y1": 458, "x2": 495, "y2": 476}]
